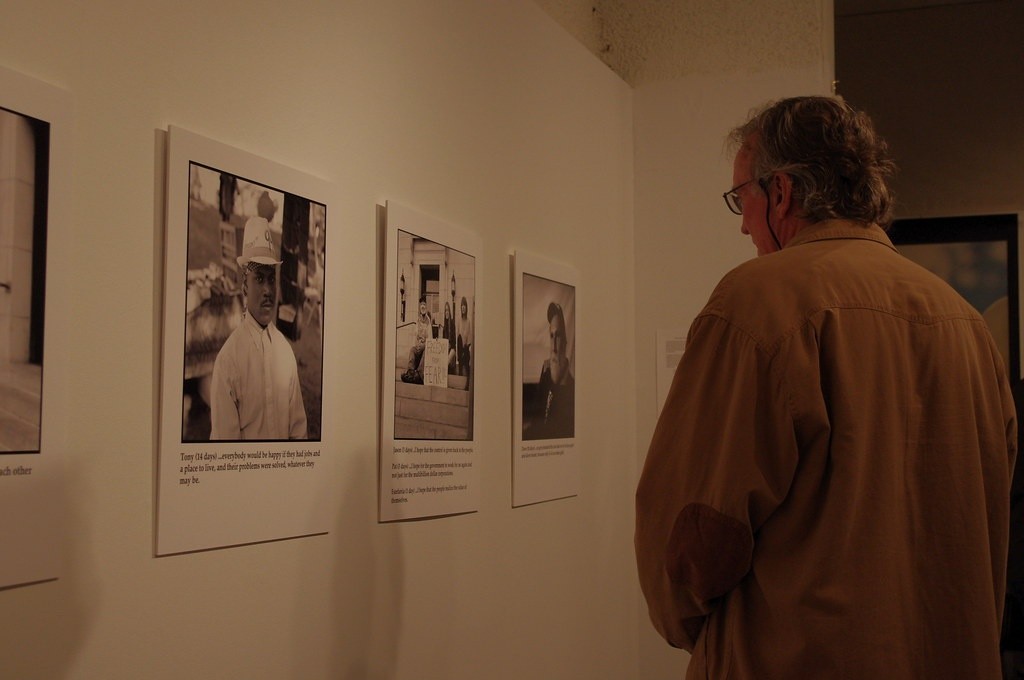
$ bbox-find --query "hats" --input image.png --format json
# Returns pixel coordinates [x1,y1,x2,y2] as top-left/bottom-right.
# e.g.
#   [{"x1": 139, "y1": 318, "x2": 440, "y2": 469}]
[
  {"x1": 546, "y1": 304, "x2": 565, "y2": 326},
  {"x1": 237, "y1": 218, "x2": 283, "y2": 267}
]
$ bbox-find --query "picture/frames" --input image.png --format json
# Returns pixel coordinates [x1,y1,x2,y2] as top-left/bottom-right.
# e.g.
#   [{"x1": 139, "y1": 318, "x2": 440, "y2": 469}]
[{"x1": 873, "y1": 213, "x2": 1019, "y2": 392}]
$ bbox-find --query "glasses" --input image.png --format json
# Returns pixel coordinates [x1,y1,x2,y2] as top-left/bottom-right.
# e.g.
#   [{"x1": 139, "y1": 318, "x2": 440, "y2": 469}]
[{"x1": 723, "y1": 173, "x2": 769, "y2": 217}]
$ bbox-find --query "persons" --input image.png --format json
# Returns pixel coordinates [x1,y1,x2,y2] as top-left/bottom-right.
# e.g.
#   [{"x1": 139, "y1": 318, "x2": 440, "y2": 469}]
[
  {"x1": 401, "y1": 297, "x2": 471, "y2": 391},
  {"x1": 211, "y1": 217, "x2": 308, "y2": 439},
  {"x1": 635, "y1": 97, "x2": 1019, "y2": 680},
  {"x1": 530, "y1": 302, "x2": 574, "y2": 439}
]
